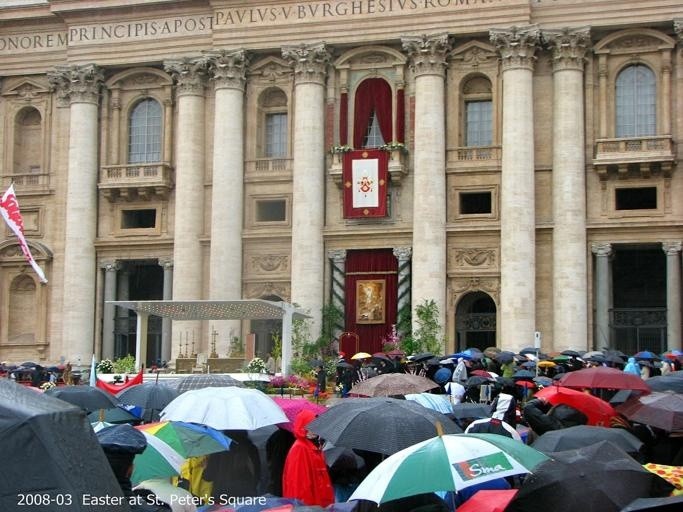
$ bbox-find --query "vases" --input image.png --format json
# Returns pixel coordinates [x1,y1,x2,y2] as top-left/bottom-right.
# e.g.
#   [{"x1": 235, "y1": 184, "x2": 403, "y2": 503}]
[
  {"x1": 102, "y1": 368, "x2": 111, "y2": 374},
  {"x1": 251, "y1": 366, "x2": 260, "y2": 373}
]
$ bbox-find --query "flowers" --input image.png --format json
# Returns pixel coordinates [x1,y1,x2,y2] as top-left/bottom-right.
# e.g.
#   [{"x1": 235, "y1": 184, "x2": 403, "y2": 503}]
[
  {"x1": 381, "y1": 141, "x2": 408, "y2": 153},
  {"x1": 247, "y1": 357, "x2": 265, "y2": 369},
  {"x1": 97, "y1": 358, "x2": 114, "y2": 371},
  {"x1": 328, "y1": 144, "x2": 352, "y2": 154}
]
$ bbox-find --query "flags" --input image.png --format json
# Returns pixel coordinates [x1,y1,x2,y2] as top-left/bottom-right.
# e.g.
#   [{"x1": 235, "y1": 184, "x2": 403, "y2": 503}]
[{"x1": 341, "y1": 149, "x2": 388, "y2": 220}]
